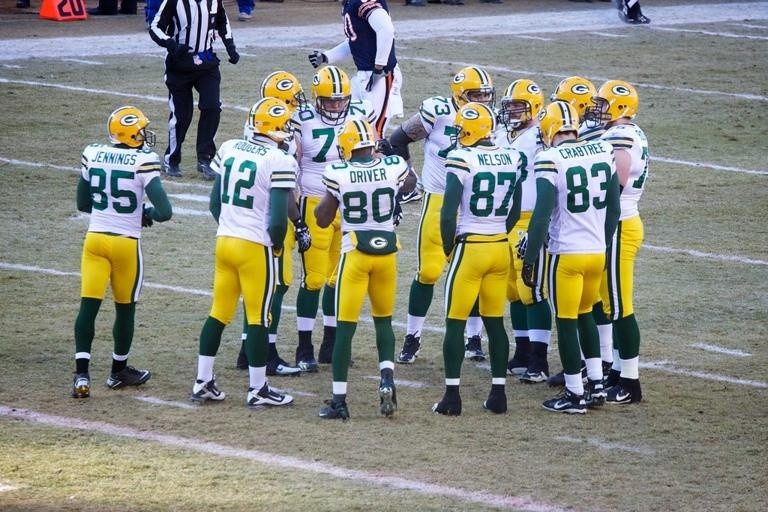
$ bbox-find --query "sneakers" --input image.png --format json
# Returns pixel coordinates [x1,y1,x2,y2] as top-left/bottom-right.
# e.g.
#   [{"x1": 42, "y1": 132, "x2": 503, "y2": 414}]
[
  {"x1": 70, "y1": 371, "x2": 91, "y2": 398},
  {"x1": 581, "y1": 360, "x2": 614, "y2": 385},
  {"x1": 583, "y1": 377, "x2": 606, "y2": 408},
  {"x1": 295, "y1": 346, "x2": 321, "y2": 373},
  {"x1": 266, "y1": 356, "x2": 302, "y2": 378},
  {"x1": 106, "y1": 365, "x2": 151, "y2": 390},
  {"x1": 518, "y1": 360, "x2": 550, "y2": 383},
  {"x1": 236, "y1": 351, "x2": 250, "y2": 369},
  {"x1": 482, "y1": 393, "x2": 508, "y2": 414},
  {"x1": 464, "y1": 335, "x2": 485, "y2": 362},
  {"x1": 237, "y1": 11, "x2": 252, "y2": 21},
  {"x1": 191, "y1": 374, "x2": 226, "y2": 403},
  {"x1": 541, "y1": 387, "x2": 588, "y2": 415},
  {"x1": 399, "y1": 180, "x2": 426, "y2": 202},
  {"x1": 431, "y1": 397, "x2": 463, "y2": 417},
  {"x1": 196, "y1": 160, "x2": 217, "y2": 181},
  {"x1": 318, "y1": 341, "x2": 355, "y2": 366},
  {"x1": 318, "y1": 399, "x2": 350, "y2": 419},
  {"x1": 397, "y1": 331, "x2": 422, "y2": 365},
  {"x1": 160, "y1": 160, "x2": 184, "y2": 177},
  {"x1": 506, "y1": 355, "x2": 528, "y2": 376},
  {"x1": 546, "y1": 359, "x2": 588, "y2": 388},
  {"x1": 246, "y1": 382, "x2": 294, "y2": 410},
  {"x1": 378, "y1": 377, "x2": 398, "y2": 417},
  {"x1": 605, "y1": 376, "x2": 643, "y2": 406}
]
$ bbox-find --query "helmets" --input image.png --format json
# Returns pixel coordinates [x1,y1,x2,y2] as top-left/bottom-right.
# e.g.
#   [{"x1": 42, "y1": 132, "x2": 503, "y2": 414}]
[
  {"x1": 258, "y1": 70, "x2": 307, "y2": 113},
  {"x1": 500, "y1": 78, "x2": 544, "y2": 133},
  {"x1": 584, "y1": 79, "x2": 640, "y2": 128},
  {"x1": 336, "y1": 119, "x2": 377, "y2": 161},
  {"x1": 550, "y1": 75, "x2": 598, "y2": 123},
  {"x1": 538, "y1": 100, "x2": 580, "y2": 151},
  {"x1": 453, "y1": 102, "x2": 496, "y2": 147},
  {"x1": 106, "y1": 105, "x2": 157, "y2": 148},
  {"x1": 310, "y1": 65, "x2": 352, "y2": 127},
  {"x1": 246, "y1": 96, "x2": 292, "y2": 142}
]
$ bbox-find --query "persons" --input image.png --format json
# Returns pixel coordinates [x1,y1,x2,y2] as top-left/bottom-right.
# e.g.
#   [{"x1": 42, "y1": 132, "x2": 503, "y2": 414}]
[
  {"x1": 550, "y1": 75, "x2": 614, "y2": 386},
  {"x1": 149, "y1": 0, "x2": 241, "y2": 180},
  {"x1": 597, "y1": 80, "x2": 649, "y2": 404},
  {"x1": 295, "y1": 65, "x2": 389, "y2": 373},
  {"x1": 70, "y1": 106, "x2": 172, "y2": 398},
  {"x1": 308, "y1": 0, "x2": 424, "y2": 203},
  {"x1": 313, "y1": 118, "x2": 418, "y2": 418},
  {"x1": 494, "y1": 80, "x2": 553, "y2": 382},
  {"x1": 389, "y1": 68, "x2": 504, "y2": 364},
  {"x1": 244, "y1": 71, "x2": 312, "y2": 376},
  {"x1": 433, "y1": 101, "x2": 522, "y2": 415},
  {"x1": 88, "y1": 0, "x2": 137, "y2": 15},
  {"x1": 523, "y1": 102, "x2": 622, "y2": 413},
  {"x1": 191, "y1": 98, "x2": 298, "y2": 407},
  {"x1": 236, "y1": 0, "x2": 256, "y2": 19}
]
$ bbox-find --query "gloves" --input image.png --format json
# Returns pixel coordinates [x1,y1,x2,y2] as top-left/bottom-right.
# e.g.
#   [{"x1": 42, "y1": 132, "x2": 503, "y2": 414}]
[
  {"x1": 520, "y1": 260, "x2": 538, "y2": 287},
  {"x1": 364, "y1": 67, "x2": 388, "y2": 92},
  {"x1": 166, "y1": 38, "x2": 192, "y2": 61},
  {"x1": 308, "y1": 50, "x2": 326, "y2": 68},
  {"x1": 514, "y1": 230, "x2": 529, "y2": 260},
  {"x1": 292, "y1": 216, "x2": 312, "y2": 253},
  {"x1": 141, "y1": 202, "x2": 154, "y2": 228},
  {"x1": 393, "y1": 197, "x2": 405, "y2": 228},
  {"x1": 223, "y1": 38, "x2": 240, "y2": 64}
]
